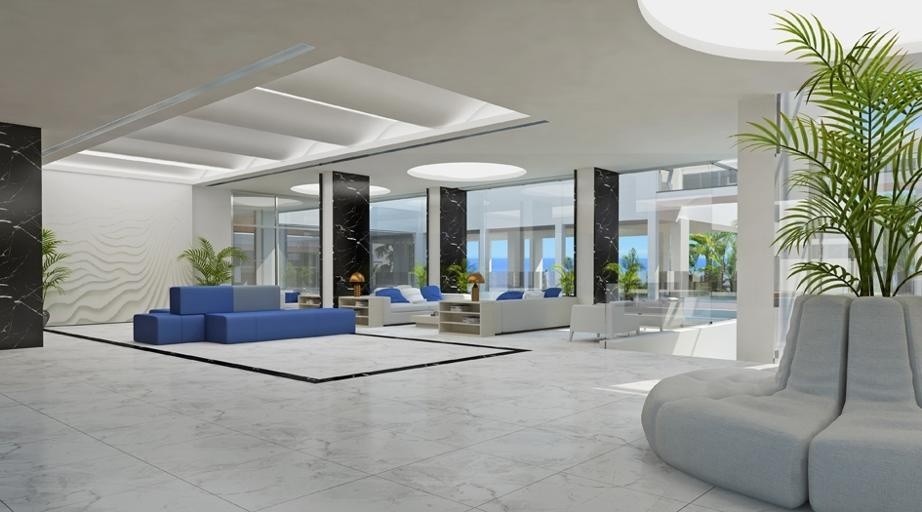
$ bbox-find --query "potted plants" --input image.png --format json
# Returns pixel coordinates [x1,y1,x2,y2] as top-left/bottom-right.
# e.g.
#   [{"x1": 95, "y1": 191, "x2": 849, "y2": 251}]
[{"x1": 41, "y1": 228, "x2": 74, "y2": 329}]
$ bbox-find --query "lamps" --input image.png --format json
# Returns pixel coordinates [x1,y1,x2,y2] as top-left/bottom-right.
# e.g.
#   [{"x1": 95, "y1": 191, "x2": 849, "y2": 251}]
[
  {"x1": 466, "y1": 272, "x2": 485, "y2": 302},
  {"x1": 349, "y1": 272, "x2": 366, "y2": 297}
]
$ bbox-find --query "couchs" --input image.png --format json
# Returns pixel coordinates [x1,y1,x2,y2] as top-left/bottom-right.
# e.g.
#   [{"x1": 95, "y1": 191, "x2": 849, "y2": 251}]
[
  {"x1": 370, "y1": 293, "x2": 471, "y2": 326},
  {"x1": 279, "y1": 289, "x2": 298, "y2": 309},
  {"x1": 494, "y1": 296, "x2": 579, "y2": 335},
  {"x1": 133, "y1": 284, "x2": 356, "y2": 346}
]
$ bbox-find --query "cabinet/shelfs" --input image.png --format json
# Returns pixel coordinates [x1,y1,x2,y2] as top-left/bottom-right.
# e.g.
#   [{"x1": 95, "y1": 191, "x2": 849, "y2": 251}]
[
  {"x1": 298, "y1": 293, "x2": 321, "y2": 308},
  {"x1": 438, "y1": 300, "x2": 496, "y2": 337},
  {"x1": 338, "y1": 295, "x2": 384, "y2": 328}
]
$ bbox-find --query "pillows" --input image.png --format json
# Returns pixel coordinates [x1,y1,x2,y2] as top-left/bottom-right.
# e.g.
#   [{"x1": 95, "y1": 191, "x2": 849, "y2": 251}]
[
  {"x1": 420, "y1": 285, "x2": 442, "y2": 302},
  {"x1": 285, "y1": 293, "x2": 298, "y2": 303},
  {"x1": 496, "y1": 290, "x2": 524, "y2": 300},
  {"x1": 375, "y1": 288, "x2": 409, "y2": 303},
  {"x1": 522, "y1": 290, "x2": 546, "y2": 300},
  {"x1": 544, "y1": 287, "x2": 563, "y2": 298},
  {"x1": 400, "y1": 287, "x2": 427, "y2": 303}
]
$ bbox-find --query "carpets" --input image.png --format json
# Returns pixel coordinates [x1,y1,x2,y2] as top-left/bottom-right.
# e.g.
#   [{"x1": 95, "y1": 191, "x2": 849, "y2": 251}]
[{"x1": 44, "y1": 322, "x2": 530, "y2": 384}]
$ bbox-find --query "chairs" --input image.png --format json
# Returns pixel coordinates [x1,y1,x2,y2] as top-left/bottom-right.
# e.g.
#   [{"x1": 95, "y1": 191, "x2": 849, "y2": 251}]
[
  {"x1": 641, "y1": 293, "x2": 921, "y2": 512},
  {"x1": 568, "y1": 303, "x2": 641, "y2": 345}
]
